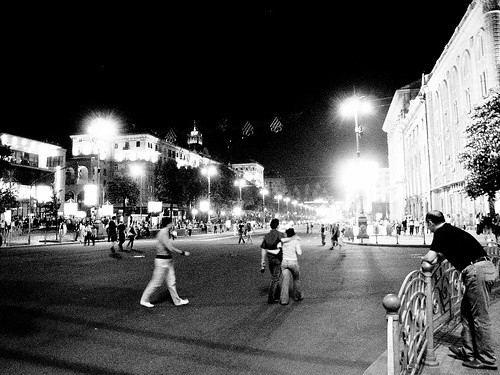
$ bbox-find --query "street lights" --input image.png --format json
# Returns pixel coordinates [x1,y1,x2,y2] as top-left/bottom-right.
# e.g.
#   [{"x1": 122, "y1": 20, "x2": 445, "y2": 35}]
[{"x1": 337, "y1": 95, "x2": 378, "y2": 239}]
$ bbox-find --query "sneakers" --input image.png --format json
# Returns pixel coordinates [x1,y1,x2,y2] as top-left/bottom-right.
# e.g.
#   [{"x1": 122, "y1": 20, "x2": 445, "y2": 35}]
[
  {"x1": 140, "y1": 299, "x2": 155, "y2": 307},
  {"x1": 175, "y1": 298, "x2": 189, "y2": 306}
]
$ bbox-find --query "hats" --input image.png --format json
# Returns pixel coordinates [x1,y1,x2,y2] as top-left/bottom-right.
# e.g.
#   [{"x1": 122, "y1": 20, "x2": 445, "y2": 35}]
[{"x1": 286, "y1": 228, "x2": 296, "y2": 238}]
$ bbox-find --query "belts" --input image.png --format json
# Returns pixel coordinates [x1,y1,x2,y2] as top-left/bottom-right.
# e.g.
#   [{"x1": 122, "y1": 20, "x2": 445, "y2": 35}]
[{"x1": 469, "y1": 258, "x2": 490, "y2": 265}]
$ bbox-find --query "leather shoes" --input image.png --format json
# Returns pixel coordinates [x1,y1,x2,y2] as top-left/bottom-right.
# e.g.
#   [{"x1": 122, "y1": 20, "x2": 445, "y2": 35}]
[
  {"x1": 462, "y1": 359, "x2": 497, "y2": 370},
  {"x1": 448, "y1": 346, "x2": 473, "y2": 360}
]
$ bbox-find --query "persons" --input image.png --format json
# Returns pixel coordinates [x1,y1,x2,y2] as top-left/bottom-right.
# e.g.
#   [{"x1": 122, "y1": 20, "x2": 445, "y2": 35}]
[
  {"x1": 58, "y1": 222, "x2": 66, "y2": 244},
  {"x1": 426, "y1": 210, "x2": 498, "y2": 370},
  {"x1": 76, "y1": 214, "x2": 206, "y2": 246},
  {"x1": 108, "y1": 216, "x2": 117, "y2": 254},
  {"x1": 0, "y1": 216, "x2": 30, "y2": 237},
  {"x1": 260, "y1": 218, "x2": 285, "y2": 304},
  {"x1": 213, "y1": 217, "x2": 258, "y2": 244},
  {"x1": 306, "y1": 220, "x2": 340, "y2": 249},
  {"x1": 126, "y1": 226, "x2": 137, "y2": 250},
  {"x1": 397, "y1": 213, "x2": 464, "y2": 236},
  {"x1": 277, "y1": 228, "x2": 305, "y2": 306},
  {"x1": 116, "y1": 219, "x2": 126, "y2": 251},
  {"x1": 140, "y1": 216, "x2": 190, "y2": 308}
]
